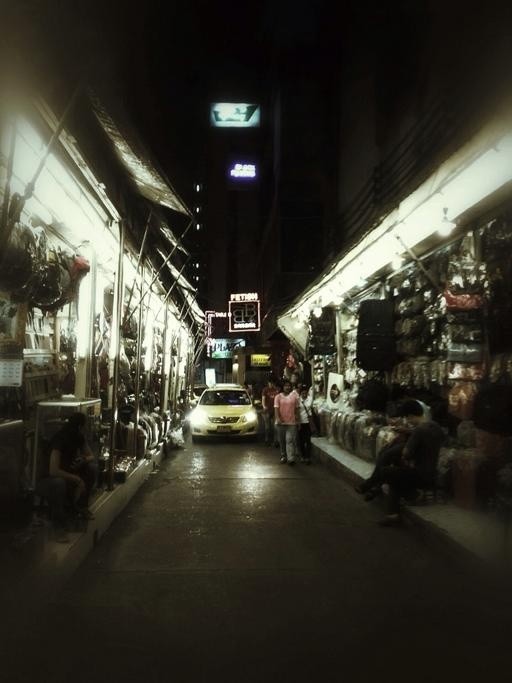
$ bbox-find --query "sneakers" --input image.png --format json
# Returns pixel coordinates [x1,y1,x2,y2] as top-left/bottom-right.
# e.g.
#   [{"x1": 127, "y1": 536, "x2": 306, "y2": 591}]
[
  {"x1": 77, "y1": 510, "x2": 95, "y2": 521},
  {"x1": 279, "y1": 455, "x2": 311, "y2": 464},
  {"x1": 56, "y1": 533, "x2": 69, "y2": 544}
]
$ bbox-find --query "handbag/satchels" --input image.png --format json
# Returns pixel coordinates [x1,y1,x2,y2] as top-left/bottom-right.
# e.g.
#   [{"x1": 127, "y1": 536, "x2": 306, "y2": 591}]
[
  {"x1": 307, "y1": 415, "x2": 318, "y2": 433},
  {"x1": 7, "y1": 224, "x2": 90, "y2": 311}
]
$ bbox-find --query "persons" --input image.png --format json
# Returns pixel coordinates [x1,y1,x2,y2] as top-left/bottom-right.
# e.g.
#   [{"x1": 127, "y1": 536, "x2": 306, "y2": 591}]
[
  {"x1": 41, "y1": 412, "x2": 96, "y2": 543},
  {"x1": 355, "y1": 400, "x2": 445, "y2": 526},
  {"x1": 244, "y1": 379, "x2": 313, "y2": 464}
]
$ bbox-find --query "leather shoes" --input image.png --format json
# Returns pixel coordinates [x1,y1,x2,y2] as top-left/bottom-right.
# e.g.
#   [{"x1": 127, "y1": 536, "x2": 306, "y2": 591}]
[{"x1": 353, "y1": 484, "x2": 404, "y2": 528}]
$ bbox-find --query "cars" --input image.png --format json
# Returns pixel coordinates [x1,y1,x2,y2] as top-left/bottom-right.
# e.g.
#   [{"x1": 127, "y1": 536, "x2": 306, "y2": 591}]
[{"x1": 190, "y1": 383, "x2": 261, "y2": 442}]
